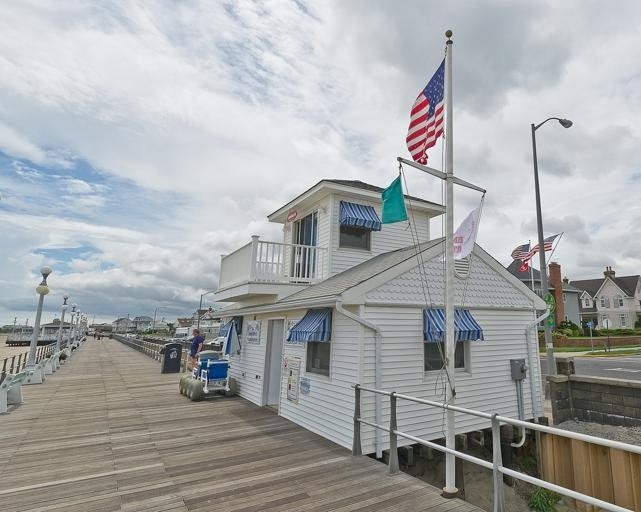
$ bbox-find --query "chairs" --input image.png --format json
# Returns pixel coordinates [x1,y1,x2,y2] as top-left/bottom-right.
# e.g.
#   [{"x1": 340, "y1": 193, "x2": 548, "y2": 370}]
[{"x1": 192, "y1": 351, "x2": 231, "y2": 394}]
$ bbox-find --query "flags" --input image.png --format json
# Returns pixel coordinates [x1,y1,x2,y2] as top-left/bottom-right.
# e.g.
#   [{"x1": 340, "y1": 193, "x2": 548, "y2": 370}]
[
  {"x1": 517, "y1": 263, "x2": 527, "y2": 272},
  {"x1": 404, "y1": 57, "x2": 447, "y2": 166},
  {"x1": 379, "y1": 172, "x2": 407, "y2": 225},
  {"x1": 437, "y1": 204, "x2": 479, "y2": 262},
  {"x1": 523, "y1": 233, "x2": 559, "y2": 261},
  {"x1": 510, "y1": 243, "x2": 529, "y2": 260}
]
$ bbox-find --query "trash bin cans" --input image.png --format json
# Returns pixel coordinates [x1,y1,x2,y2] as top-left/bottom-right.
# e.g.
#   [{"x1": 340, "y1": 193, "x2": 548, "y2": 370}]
[{"x1": 159, "y1": 343, "x2": 182, "y2": 373}]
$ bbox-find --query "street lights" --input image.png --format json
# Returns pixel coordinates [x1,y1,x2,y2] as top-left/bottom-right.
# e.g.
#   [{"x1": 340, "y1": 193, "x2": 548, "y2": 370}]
[
  {"x1": 56, "y1": 293, "x2": 69, "y2": 352},
  {"x1": 66, "y1": 303, "x2": 88, "y2": 348},
  {"x1": 24, "y1": 267, "x2": 52, "y2": 370},
  {"x1": 12, "y1": 317, "x2": 16, "y2": 334},
  {"x1": 529, "y1": 117, "x2": 573, "y2": 376}
]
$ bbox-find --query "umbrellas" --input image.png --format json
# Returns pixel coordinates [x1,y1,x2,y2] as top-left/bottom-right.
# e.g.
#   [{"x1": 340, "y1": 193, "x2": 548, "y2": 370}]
[{"x1": 219, "y1": 319, "x2": 241, "y2": 388}]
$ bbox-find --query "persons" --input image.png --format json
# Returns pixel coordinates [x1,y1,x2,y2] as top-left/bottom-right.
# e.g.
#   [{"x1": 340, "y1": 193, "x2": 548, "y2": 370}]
[{"x1": 189, "y1": 328, "x2": 203, "y2": 367}]
